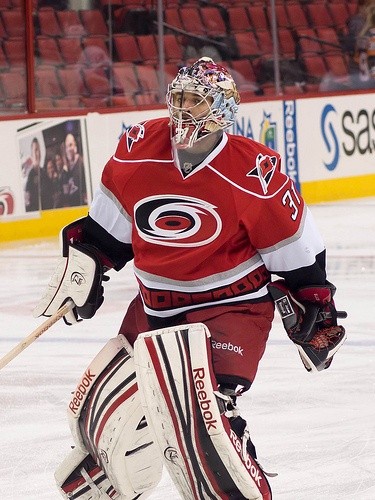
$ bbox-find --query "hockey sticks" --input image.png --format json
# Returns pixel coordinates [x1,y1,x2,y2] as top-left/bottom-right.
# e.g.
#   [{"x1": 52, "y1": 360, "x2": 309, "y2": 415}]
[{"x1": 0, "y1": 296, "x2": 79, "y2": 372}]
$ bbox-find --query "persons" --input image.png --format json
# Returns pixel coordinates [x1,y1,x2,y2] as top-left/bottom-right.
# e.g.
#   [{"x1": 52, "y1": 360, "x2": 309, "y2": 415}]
[
  {"x1": 33, "y1": 57, "x2": 347, "y2": 500},
  {"x1": 340, "y1": 0, "x2": 375, "y2": 88},
  {"x1": 21, "y1": 133, "x2": 89, "y2": 212}
]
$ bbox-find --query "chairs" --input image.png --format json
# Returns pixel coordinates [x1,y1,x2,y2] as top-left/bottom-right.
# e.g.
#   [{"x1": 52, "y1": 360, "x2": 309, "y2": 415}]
[{"x1": 0, "y1": 0, "x2": 375, "y2": 115}]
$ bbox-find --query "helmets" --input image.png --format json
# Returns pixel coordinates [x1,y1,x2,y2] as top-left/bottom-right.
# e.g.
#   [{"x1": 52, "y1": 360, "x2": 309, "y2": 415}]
[{"x1": 168, "y1": 57, "x2": 240, "y2": 149}]
